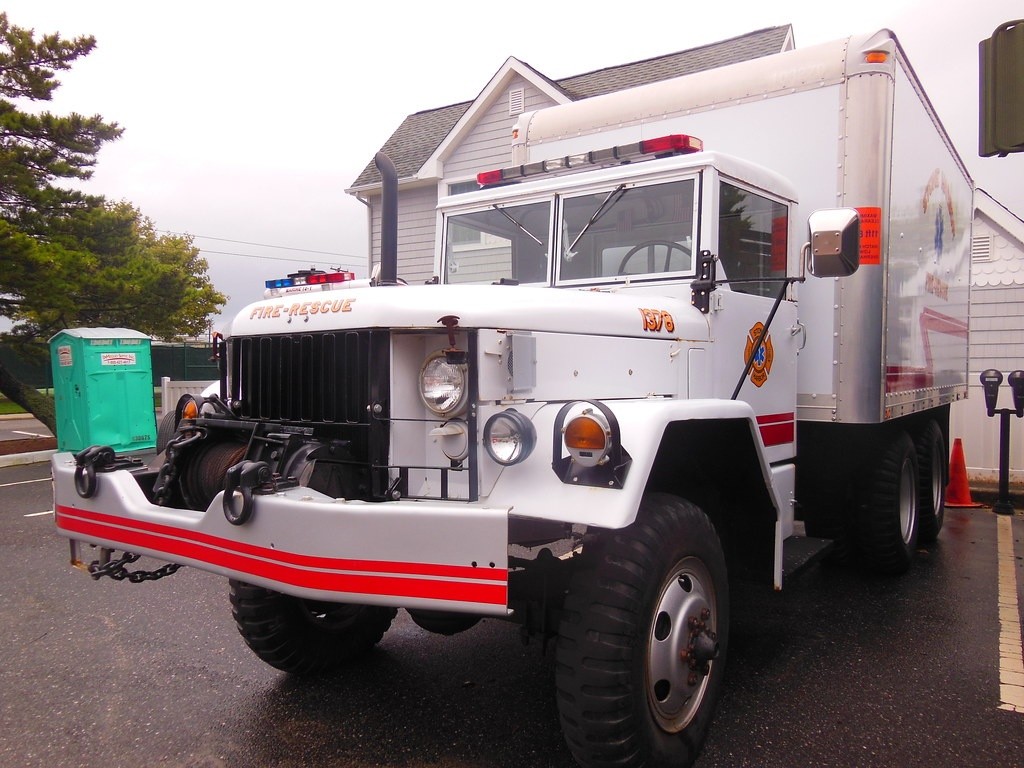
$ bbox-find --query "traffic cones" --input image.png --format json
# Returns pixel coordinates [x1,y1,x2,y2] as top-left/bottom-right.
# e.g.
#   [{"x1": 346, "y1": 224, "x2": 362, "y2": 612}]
[{"x1": 944, "y1": 438, "x2": 981, "y2": 508}]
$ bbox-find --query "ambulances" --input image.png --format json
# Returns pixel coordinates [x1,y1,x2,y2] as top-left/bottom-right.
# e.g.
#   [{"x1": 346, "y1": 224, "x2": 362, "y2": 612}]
[{"x1": 55, "y1": 27, "x2": 976, "y2": 768}]
